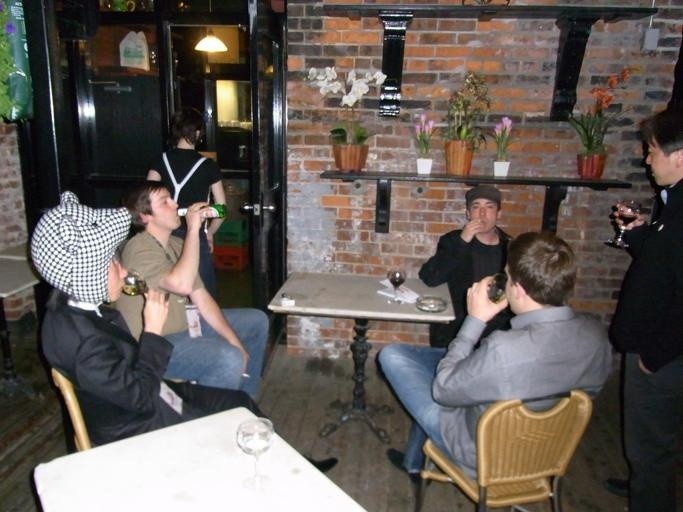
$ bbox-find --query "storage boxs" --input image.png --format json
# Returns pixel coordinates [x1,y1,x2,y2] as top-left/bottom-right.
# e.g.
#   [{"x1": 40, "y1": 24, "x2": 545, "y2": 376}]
[
  {"x1": 213, "y1": 218, "x2": 249, "y2": 246},
  {"x1": 214, "y1": 246, "x2": 249, "y2": 271}
]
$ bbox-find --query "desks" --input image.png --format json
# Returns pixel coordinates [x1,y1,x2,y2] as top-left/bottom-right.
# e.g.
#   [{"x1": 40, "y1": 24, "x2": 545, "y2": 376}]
[{"x1": 0, "y1": 244, "x2": 40, "y2": 395}]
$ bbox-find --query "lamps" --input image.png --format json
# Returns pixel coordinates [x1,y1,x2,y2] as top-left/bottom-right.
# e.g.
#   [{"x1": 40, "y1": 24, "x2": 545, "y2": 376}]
[{"x1": 195, "y1": 0, "x2": 228, "y2": 52}]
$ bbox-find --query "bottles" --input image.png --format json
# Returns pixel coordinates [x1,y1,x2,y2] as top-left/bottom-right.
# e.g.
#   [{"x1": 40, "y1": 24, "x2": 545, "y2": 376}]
[{"x1": 179, "y1": 205, "x2": 227, "y2": 219}]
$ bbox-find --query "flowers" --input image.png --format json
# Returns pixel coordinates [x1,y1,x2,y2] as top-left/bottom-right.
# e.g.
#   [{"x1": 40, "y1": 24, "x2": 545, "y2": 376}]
[
  {"x1": 414, "y1": 115, "x2": 434, "y2": 159},
  {"x1": 494, "y1": 118, "x2": 513, "y2": 161},
  {"x1": 308, "y1": 67, "x2": 387, "y2": 144},
  {"x1": 567, "y1": 66, "x2": 645, "y2": 158},
  {"x1": 440, "y1": 71, "x2": 494, "y2": 151}
]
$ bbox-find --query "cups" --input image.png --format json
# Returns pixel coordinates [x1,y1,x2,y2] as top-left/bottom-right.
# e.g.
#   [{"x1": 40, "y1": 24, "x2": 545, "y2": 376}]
[{"x1": 487, "y1": 273, "x2": 508, "y2": 304}]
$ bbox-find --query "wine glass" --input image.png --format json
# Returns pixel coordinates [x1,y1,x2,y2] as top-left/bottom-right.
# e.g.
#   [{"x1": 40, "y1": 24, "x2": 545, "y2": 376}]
[
  {"x1": 121, "y1": 269, "x2": 171, "y2": 304},
  {"x1": 606, "y1": 200, "x2": 642, "y2": 250},
  {"x1": 386, "y1": 267, "x2": 408, "y2": 305}
]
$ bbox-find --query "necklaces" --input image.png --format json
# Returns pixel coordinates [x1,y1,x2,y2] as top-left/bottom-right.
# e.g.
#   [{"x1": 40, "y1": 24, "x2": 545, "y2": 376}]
[{"x1": 151, "y1": 233, "x2": 179, "y2": 266}]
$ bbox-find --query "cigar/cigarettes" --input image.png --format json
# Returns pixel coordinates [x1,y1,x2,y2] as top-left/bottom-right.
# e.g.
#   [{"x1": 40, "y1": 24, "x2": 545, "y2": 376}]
[{"x1": 243, "y1": 372, "x2": 251, "y2": 379}]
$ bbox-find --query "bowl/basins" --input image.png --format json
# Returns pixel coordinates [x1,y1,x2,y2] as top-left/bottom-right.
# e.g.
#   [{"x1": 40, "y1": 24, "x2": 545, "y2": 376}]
[{"x1": 417, "y1": 296, "x2": 451, "y2": 312}]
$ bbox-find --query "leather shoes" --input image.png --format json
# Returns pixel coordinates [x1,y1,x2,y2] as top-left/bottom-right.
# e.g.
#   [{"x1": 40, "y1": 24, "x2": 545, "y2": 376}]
[
  {"x1": 298, "y1": 451, "x2": 338, "y2": 474},
  {"x1": 384, "y1": 443, "x2": 431, "y2": 492}
]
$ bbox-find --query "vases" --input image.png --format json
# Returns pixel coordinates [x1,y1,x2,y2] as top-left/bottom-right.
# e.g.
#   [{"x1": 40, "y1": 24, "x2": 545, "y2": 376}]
[
  {"x1": 417, "y1": 159, "x2": 432, "y2": 178},
  {"x1": 494, "y1": 161, "x2": 510, "y2": 176},
  {"x1": 446, "y1": 141, "x2": 473, "y2": 175},
  {"x1": 577, "y1": 155, "x2": 606, "y2": 180},
  {"x1": 333, "y1": 144, "x2": 368, "y2": 173}
]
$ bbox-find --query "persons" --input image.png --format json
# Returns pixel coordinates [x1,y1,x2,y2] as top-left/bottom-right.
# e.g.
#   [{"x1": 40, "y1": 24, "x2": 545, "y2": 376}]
[
  {"x1": 418, "y1": 185, "x2": 517, "y2": 349},
  {"x1": 603, "y1": 111, "x2": 683, "y2": 511},
  {"x1": 40, "y1": 259, "x2": 339, "y2": 472},
  {"x1": 112, "y1": 180, "x2": 270, "y2": 402},
  {"x1": 147, "y1": 107, "x2": 228, "y2": 303},
  {"x1": 377, "y1": 230, "x2": 614, "y2": 486}
]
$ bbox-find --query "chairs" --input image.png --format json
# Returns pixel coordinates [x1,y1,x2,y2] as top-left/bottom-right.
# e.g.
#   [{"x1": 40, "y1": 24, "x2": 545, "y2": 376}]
[
  {"x1": 51, "y1": 368, "x2": 91, "y2": 451},
  {"x1": 422, "y1": 389, "x2": 592, "y2": 512}
]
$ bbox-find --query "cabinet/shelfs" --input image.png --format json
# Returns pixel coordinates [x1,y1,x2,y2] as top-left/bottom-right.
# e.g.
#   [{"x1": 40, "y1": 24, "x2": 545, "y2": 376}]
[{"x1": 320, "y1": 4, "x2": 658, "y2": 236}]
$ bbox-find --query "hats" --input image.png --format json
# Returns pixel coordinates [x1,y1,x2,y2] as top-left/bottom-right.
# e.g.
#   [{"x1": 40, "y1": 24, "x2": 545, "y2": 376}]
[
  {"x1": 29, "y1": 190, "x2": 132, "y2": 306},
  {"x1": 462, "y1": 184, "x2": 502, "y2": 207}
]
